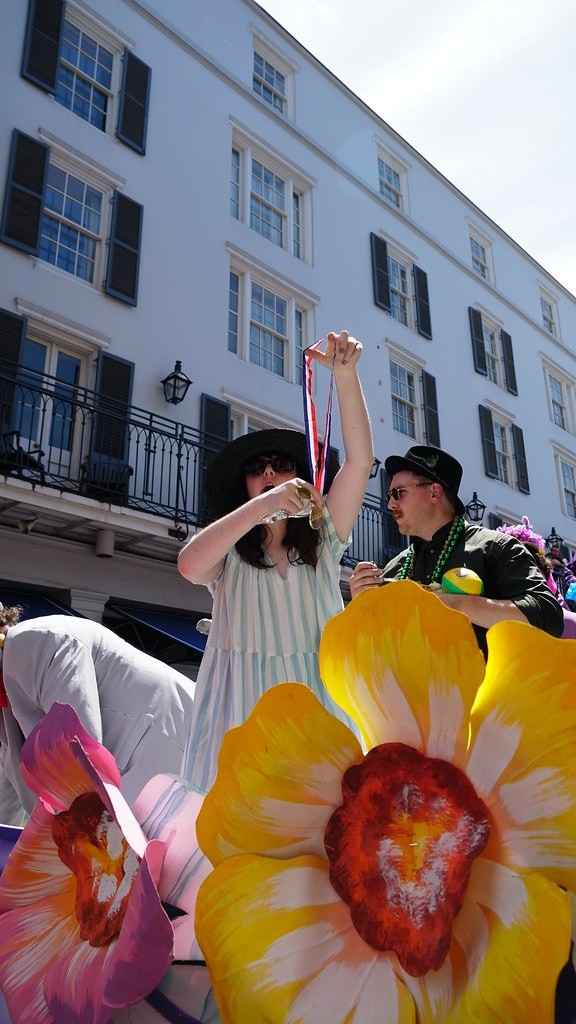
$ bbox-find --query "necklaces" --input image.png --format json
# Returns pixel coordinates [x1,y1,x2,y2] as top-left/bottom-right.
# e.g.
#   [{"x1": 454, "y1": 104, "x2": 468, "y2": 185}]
[{"x1": 400, "y1": 515, "x2": 464, "y2": 584}]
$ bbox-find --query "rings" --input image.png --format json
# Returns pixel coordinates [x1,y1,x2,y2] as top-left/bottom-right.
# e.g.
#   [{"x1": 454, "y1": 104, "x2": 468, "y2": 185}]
[{"x1": 297, "y1": 486, "x2": 312, "y2": 500}]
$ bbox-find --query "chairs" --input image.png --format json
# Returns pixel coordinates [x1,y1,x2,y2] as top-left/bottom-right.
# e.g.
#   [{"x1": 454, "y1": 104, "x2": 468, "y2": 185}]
[
  {"x1": 78, "y1": 452, "x2": 133, "y2": 506},
  {"x1": 0, "y1": 430, "x2": 47, "y2": 485}
]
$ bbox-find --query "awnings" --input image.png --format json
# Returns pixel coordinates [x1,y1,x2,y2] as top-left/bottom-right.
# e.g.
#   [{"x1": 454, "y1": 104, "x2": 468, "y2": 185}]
[
  {"x1": 0, "y1": 590, "x2": 88, "y2": 623},
  {"x1": 109, "y1": 605, "x2": 207, "y2": 653}
]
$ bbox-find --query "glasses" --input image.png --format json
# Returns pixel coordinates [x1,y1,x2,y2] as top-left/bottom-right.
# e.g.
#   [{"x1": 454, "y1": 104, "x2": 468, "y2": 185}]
[
  {"x1": 386, "y1": 481, "x2": 429, "y2": 500},
  {"x1": 244, "y1": 453, "x2": 298, "y2": 475}
]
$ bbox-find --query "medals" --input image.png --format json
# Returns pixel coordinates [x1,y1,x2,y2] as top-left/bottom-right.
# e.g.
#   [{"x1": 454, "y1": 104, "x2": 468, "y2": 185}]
[{"x1": 309, "y1": 506, "x2": 325, "y2": 529}]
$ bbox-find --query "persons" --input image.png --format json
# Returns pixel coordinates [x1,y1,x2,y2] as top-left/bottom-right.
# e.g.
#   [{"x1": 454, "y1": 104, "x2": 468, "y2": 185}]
[
  {"x1": 495, "y1": 516, "x2": 576, "y2": 612},
  {"x1": 179, "y1": 330, "x2": 374, "y2": 793},
  {"x1": 350, "y1": 447, "x2": 565, "y2": 663},
  {"x1": 0, "y1": 603, "x2": 196, "y2": 828}
]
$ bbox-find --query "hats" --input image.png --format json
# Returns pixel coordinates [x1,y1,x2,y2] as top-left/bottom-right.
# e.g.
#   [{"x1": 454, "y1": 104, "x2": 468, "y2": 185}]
[
  {"x1": 210, "y1": 428, "x2": 307, "y2": 485},
  {"x1": 385, "y1": 445, "x2": 465, "y2": 513}
]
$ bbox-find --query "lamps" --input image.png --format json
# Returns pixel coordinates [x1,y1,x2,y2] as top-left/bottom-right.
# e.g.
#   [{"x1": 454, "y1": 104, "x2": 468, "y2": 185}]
[
  {"x1": 465, "y1": 491, "x2": 487, "y2": 522},
  {"x1": 160, "y1": 361, "x2": 193, "y2": 403},
  {"x1": 370, "y1": 456, "x2": 382, "y2": 479},
  {"x1": 544, "y1": 527, "x2": 564, "y2": 551}
]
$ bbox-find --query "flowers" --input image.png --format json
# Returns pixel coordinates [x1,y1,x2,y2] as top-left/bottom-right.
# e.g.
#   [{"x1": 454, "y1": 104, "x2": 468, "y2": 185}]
[
  {"x1": 0, "y1": 700, "x2": 174, "y2": 1024},
  {"x1": 196, "y1": 576, "x2": 576, "y2": 1023}
]
